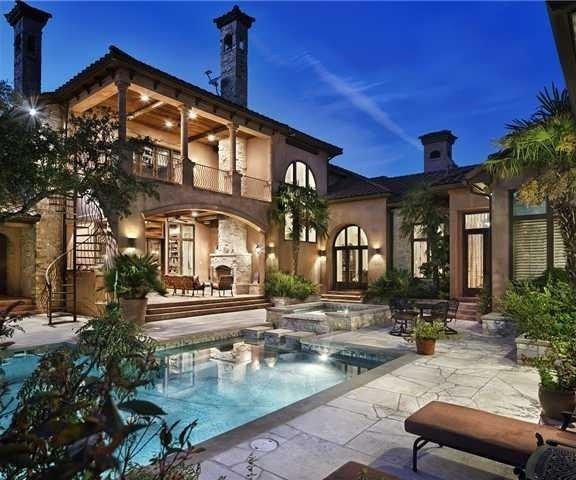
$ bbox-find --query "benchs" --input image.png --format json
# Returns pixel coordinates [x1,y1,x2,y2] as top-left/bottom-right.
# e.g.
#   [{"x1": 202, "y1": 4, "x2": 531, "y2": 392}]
[{"x1": 405, "y1": 400, "x2": 574, "y2": 473}]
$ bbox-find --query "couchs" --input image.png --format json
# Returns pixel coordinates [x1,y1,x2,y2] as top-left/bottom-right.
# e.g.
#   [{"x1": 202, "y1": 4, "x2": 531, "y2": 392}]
[{"x1": 161, "y1": 274, "x2": 205, "y2": 296}]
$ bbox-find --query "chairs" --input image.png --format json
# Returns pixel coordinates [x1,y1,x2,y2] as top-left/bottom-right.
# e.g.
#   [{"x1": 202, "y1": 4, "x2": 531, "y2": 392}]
[
  {"x1": 211, "y1": 275, "x2": 234, "y2": 297},
  {"x1": 389, "y1": 298, "x2": 459, "y2": 336}
]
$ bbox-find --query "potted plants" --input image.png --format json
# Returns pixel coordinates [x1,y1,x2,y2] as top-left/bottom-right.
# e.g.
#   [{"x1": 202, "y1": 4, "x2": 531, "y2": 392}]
[
  {"x1": 263, "y1": 270, "x2": 323, "y2": 308},
  {"x1": 403, "y1": 316, "x2": 448, "y2": 355},
  {"x1": 520, "y1": 334, "x2": 576, "y2": 421},
  {"x1": 92, "y1": 251, "x2": 169, "y2": 326}
]
